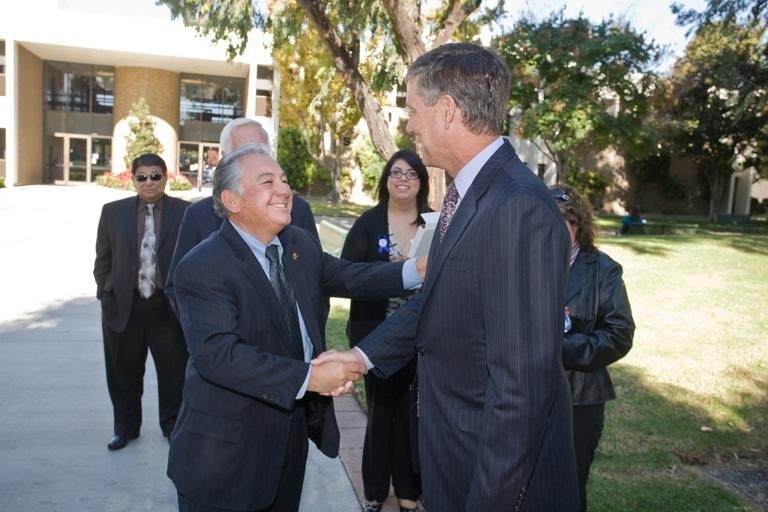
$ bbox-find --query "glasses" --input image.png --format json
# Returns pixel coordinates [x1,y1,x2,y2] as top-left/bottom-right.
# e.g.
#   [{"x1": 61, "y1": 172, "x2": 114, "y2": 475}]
[
  {"x1": 135, "y1": 173, "x2": 161, "y2": 181},
  {"x1": 549, "y1": 189, "x2": 569, "y2": 202},
  {"x1": 388, "y1": 170, "x2": 417, "y2": 179}
]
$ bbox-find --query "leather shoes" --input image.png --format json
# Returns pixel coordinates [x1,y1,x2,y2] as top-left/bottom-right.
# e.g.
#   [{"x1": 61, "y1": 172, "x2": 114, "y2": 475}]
[
  {"x1": 396, "y1": 499, "x2": 418, "y2": 512},
  {"x1": 107, "y1": 433, "x2": 139, "y2": 450},
  {"x1": 362, "y1": 500, "x2": 381, "y2": 512}
]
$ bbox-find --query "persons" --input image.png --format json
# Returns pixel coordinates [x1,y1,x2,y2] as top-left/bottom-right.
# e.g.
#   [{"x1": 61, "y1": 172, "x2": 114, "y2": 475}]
[
  {"x1": 93, "y1": 153, "x2": 193, "y2": 450},
  {"x1": 167, "y1": 142, "x2": 429, "y2": 512},
  {"x1": 548, "y1": 184, "x2": 636, "y2": 512},
  {"x1": 164, "y1": 117, "x2": 319, "y2": 333},
  {"x1": 309, "y1": 43, "x2": 581, "y2": 512},
  {"x1": 339, "y1": 148, "x2": 436, "y2": 512}
]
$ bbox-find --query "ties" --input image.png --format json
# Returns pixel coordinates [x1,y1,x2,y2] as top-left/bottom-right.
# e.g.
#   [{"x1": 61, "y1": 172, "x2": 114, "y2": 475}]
[
  {"x1": 265, "y1": 245, "x2": 303, "y2": 362},
  {"x1": 439, "y1": 180, "x2": 458, "y2": 244},
  {"x1": 138, "y1": 204, "x2": 157, "y2": 300}
]
